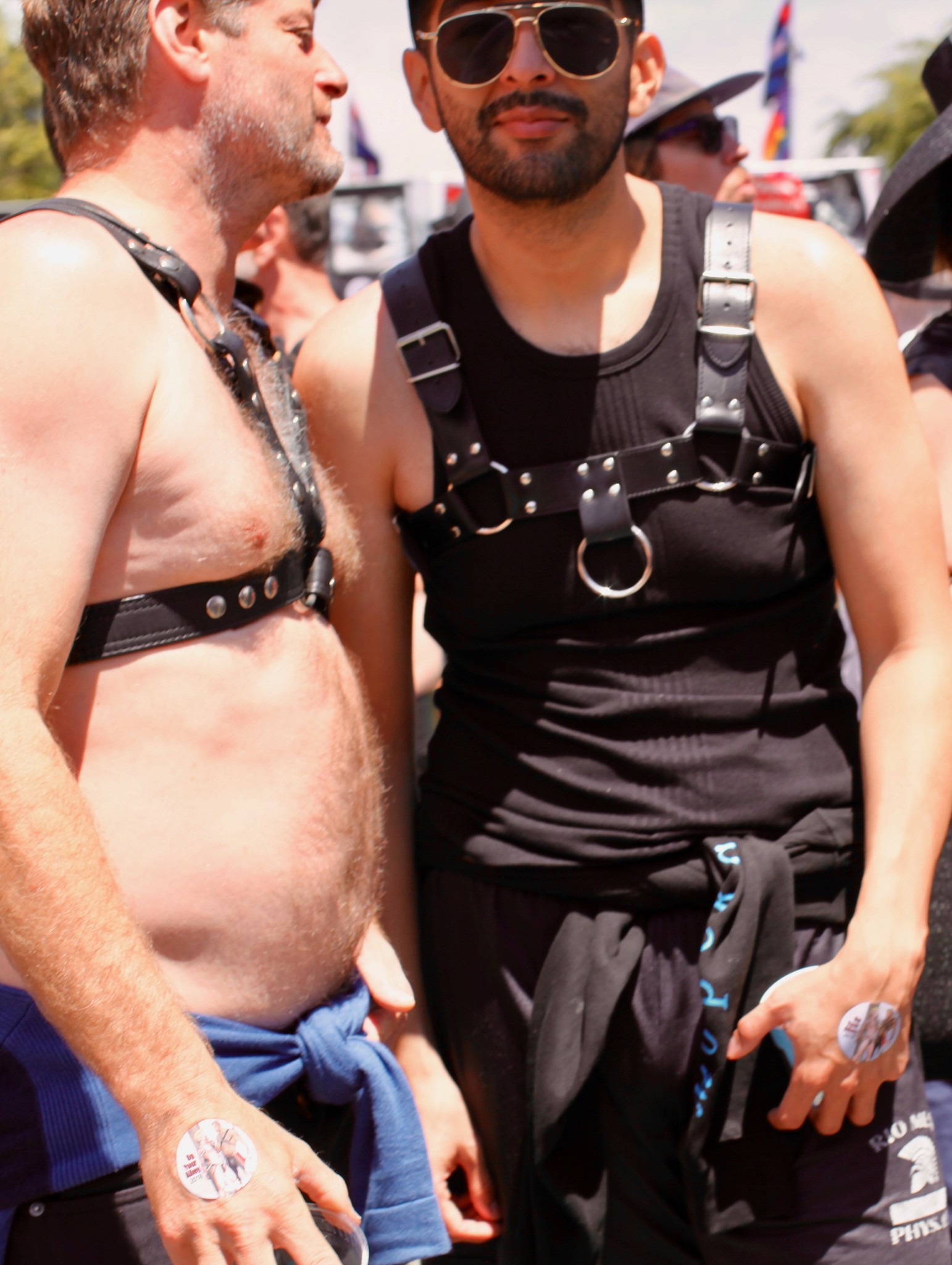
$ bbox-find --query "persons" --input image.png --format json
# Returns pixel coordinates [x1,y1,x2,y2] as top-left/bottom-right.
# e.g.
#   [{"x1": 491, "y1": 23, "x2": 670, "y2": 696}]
[
  {"x1": 290, "y1": 1, "x2": 951, "y2": 1265},
  {"x1": 621, "y1": 64, "x2": 766, "y2": 206},
  {"x1": 820, "y1": 35, "x2": 949, "y2": 1147},
  {"x1": 0, "y1": 0, "x2": 457, "y2": 1265},
  {"x1": 231, "y1": 192, "x2": 339, "y2": 373}
]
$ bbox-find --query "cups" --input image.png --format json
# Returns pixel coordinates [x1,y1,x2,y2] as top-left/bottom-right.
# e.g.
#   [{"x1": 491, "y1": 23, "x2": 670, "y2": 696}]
[{"x1": 274, "y1": 1204, "x2": 369, "y2": 1265}]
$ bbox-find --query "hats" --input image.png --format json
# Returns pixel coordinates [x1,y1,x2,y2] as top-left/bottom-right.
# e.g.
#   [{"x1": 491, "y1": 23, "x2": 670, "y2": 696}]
[
  {"x1": 858, "y1": 29, "x2": 952, "y2": 299},
  {"x1": 622, "y1": 64, "x2": 766, "y2": 139}
]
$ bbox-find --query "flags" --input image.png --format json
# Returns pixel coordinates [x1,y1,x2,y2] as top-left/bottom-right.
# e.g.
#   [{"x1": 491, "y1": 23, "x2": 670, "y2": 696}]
[
  {"x1": 762, "y1": 0, "x2": 792, "y2": 102},
  {"x1": 765, "y1": 104, "x2": 788, "y2": 162},
  {"x1": 751, "y1": 172, "x2": 812, "y2": 220}
]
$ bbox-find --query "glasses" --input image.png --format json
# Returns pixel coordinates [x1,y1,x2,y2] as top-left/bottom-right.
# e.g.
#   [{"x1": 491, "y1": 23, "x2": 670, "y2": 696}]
[
  {"x1": 655, "y1": 114, "x2": 724, "y2": 154},
  {"x1": 412, "y1": 2, "x2": 641, "y2": 89}
]
